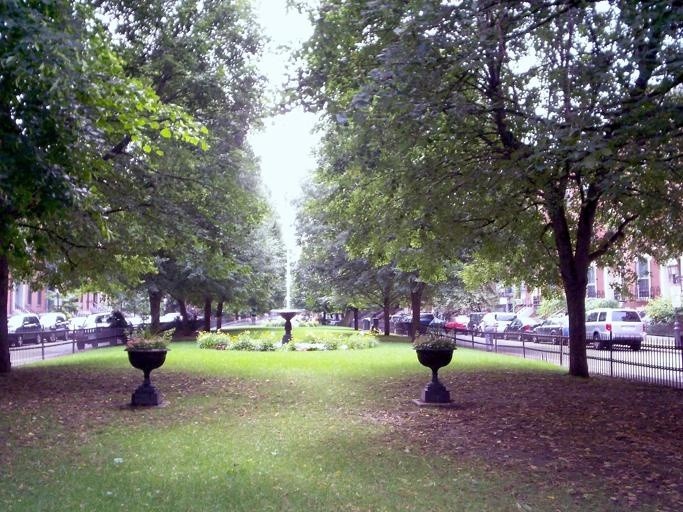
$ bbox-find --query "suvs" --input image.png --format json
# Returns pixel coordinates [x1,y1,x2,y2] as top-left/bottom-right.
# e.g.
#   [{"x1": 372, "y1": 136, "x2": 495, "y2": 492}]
[
  {"x1": 75, "y1": 313, "x2": 120, "y2": 351},
  {"x1": 561, "y1": 308, "x2": 645, "y2": 351},
  {"x1": 38, "y1": 312, "x2": 69, "y2": 342},
  {"x1": 7, "y1": 312, "x2": 42, "y2": 347},
  {"x1": 477, "y1": 312, "x2": 517, "y2": 339}
]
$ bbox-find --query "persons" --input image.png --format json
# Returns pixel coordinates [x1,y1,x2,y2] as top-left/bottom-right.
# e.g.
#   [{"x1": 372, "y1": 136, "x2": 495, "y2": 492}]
[
  {"x1": 509, "y1": 315, "x2": 523, "y2": 330},
  {"x1": 316, "y1": 314, "x2": 323, "y2": 324},
  {"x1": 481, "y1": 307, "x2": 499, "y2": 352}
]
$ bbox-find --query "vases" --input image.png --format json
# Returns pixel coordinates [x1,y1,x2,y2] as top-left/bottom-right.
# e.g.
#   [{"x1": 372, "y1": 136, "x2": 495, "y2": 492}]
[
  {"x1": 124, "y1": 347, "x2": 167, "y2": 407},
  {"x1": 413, "y1": 347, "x2": 456, "y2": 403}
]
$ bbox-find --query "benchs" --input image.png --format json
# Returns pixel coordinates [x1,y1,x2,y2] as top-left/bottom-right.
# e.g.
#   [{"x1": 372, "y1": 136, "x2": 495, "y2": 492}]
[{"x1": 74, "y1": 322, "x2": 126, "y2": 350}]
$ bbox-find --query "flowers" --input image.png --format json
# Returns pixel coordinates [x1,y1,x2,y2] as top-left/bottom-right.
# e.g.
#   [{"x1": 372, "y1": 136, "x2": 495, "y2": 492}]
[
  {"x1": 121, "y1": 314, "x2": 174, "y2": 349},
  {"x1": 414, "y1": 325, "x2": 456, "y2": 349}
]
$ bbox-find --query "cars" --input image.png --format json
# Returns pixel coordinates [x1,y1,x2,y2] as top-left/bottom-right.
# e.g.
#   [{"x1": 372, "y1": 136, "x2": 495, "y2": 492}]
[
  {"x1": 67, "y1": 317, "x2": 89, "y2": 339},
  {"x1": 504, "y1": 317, "x2": 537, "y2": 341},
  {"x1": 531, "y1": 316, "x2": 566, "y2": 345},
  {"x1": 378, "y1": 310, "x2": 483, "y2": 338}
]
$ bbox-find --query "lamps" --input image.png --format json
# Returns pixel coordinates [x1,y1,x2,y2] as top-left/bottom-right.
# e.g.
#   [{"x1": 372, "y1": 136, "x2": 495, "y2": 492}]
[{"x1": 665, "y1": 259, "x2": 682, "y2": 284}]
